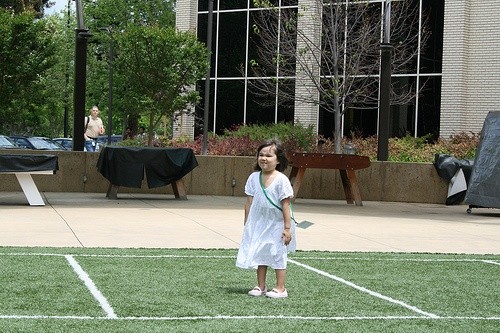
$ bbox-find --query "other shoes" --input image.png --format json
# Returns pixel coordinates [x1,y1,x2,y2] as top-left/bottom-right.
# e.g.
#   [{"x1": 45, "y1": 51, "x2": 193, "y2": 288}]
[
  {"x1": 266, "y1": 288, "x2": 288, "y2": 298},
  {"x1": 248, "y1": 286, "x2": 267, "y2": 296}
]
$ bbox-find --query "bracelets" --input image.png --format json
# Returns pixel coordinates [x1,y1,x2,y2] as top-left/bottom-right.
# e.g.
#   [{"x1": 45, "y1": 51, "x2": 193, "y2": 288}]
[{"x1": 285, "y1": 228, "x2": 290, "y2": 230}]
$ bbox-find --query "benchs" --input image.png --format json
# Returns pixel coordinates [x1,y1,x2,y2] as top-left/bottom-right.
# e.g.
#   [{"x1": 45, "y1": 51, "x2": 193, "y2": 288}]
[
  {"x1": 286, "y1": 150, "x2": 372, "y2": 208},
  {"x1": 0, "y1": 153, "x2": 60, "y2": 206},
  {"x1": 94, "y1": 145, "x2": 197, "y2": 202}
]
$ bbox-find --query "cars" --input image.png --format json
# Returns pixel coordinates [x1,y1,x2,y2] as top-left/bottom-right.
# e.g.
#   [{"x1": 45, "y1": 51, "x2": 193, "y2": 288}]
[
  {"x1": 0, "y1": 134, "x2": 62, "y2": 151},
  {"x1": 52, "y1": 135, "x2": 123, "y2": 151}
]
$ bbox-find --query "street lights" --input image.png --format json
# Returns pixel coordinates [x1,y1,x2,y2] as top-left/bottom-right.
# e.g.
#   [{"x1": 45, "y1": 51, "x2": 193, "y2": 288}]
[{"x1": 99, "y1": 26, "x2": 113, "y2": 146}]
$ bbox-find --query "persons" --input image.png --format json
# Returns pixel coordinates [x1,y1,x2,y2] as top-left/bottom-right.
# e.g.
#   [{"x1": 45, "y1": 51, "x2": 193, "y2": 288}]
[
  {"x1": 84, "y1": 105, "x2": 104, "y2": 152},
  {"x1": 124, "y1": 127, "x2": 132, "y2": 140},
  {"x1": 235, "y1": 139, "x2": 296, "y2": 297},
  {"x1": 137, "y1": 126, "x2": 146, "y2": 138}
]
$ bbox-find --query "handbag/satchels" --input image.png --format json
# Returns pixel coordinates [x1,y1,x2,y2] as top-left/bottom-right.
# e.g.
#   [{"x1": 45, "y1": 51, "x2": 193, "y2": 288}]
[{"x1": 290, "y1": 199, "x2": 294, "y2": 219}]
